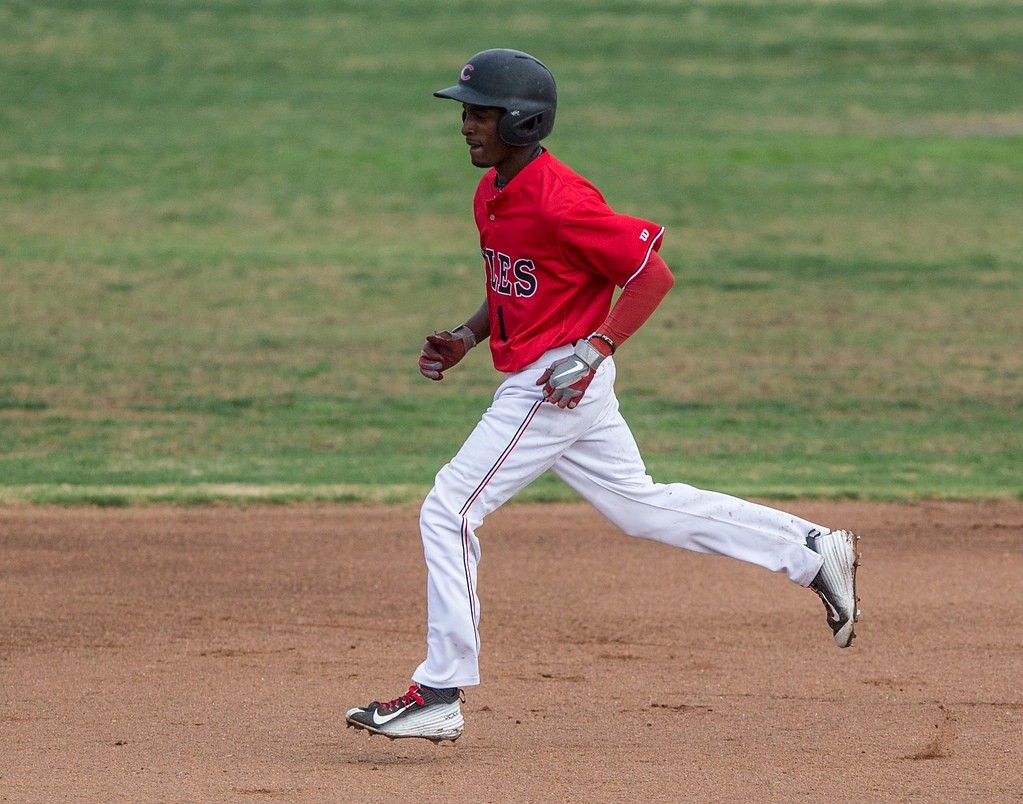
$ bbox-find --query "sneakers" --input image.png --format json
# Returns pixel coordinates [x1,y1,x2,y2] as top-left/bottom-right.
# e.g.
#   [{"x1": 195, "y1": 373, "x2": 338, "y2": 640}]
[
  {"x1": 805, "y1": 529, "x2": 861, "y2": 648},
  {"x1": 343, "y1": 682, "x2": 464, "y2": 746}
]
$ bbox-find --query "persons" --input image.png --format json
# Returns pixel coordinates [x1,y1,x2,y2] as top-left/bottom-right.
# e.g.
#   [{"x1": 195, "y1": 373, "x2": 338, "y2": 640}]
[{"x1": 345, "y1": 48, "x2": 864, "y2": 746}]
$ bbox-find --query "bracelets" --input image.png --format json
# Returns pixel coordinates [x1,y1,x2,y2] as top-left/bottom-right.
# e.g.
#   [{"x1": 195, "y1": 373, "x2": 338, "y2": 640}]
[{"x1": 588, "y1": 335, "x2": 617, "y2": 356}]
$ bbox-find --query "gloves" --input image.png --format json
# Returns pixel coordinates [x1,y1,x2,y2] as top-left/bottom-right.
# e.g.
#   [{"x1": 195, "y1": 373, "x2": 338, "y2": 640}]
[
  {"x1": 535, "y1": 338, "x2": 606, "y2": 409},
  {"x1": 418, "y1": 322, "x2": 476, "y2": 381}
]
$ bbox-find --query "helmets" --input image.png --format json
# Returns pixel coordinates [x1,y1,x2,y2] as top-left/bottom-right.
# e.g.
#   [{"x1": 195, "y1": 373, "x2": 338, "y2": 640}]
[{"x1": 433, "y1": 48, "x2": 557, "y2": 146}]
